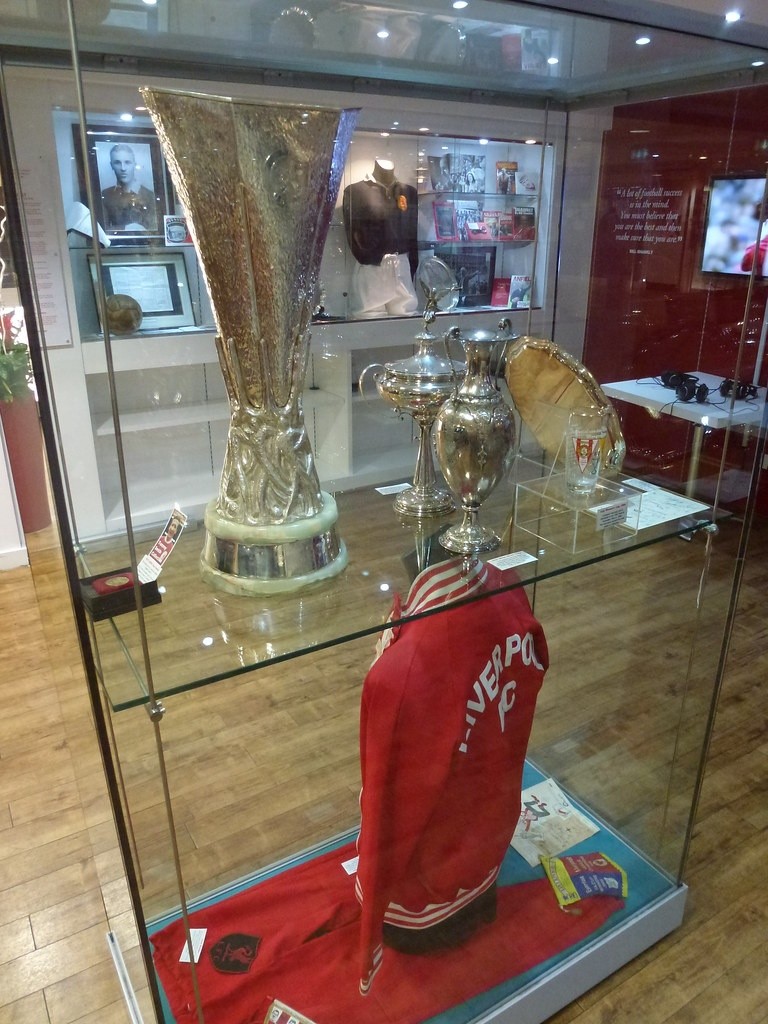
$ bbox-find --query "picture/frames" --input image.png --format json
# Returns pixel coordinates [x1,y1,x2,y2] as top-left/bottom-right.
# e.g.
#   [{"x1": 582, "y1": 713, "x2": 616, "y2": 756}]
[
  {"x1": 73, "y1": 123, "x2": 174, "y2": 245},
  {"x1": 433, "y1": 202, "x2": 459, "y2": 241}
]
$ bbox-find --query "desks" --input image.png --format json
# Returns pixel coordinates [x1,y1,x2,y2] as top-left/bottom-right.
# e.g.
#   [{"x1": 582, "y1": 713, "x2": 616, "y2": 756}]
[{"x1": 600, "y1": 372, "x2": 768, "y2": 542}]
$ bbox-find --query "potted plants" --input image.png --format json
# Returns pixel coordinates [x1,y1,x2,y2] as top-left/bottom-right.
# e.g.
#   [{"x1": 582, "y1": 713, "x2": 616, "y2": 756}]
[{"x1": 0, "y1": 320, "x2": 52, "y2": 535}]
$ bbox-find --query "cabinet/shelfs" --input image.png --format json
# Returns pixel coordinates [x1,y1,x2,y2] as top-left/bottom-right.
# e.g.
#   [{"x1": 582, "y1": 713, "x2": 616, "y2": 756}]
[
  {"x1": 1, "y1": 62, "x2": 570, "y2": 545},
  {"x1": 0, "y1": 0, "x2": 768, "y2": 1024}
]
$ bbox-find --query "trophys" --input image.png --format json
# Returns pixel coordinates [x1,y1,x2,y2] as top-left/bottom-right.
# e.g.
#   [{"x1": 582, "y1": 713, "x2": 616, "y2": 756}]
[
  {"x1": 358, "y1": 306, "x2": 465, "y2": 518},
  {"x1": 137, "y1": 86, "x2": 361, "y2": 593},
  {"x1": 432, "y1": 318, "x2": 516, "y2": 554}
]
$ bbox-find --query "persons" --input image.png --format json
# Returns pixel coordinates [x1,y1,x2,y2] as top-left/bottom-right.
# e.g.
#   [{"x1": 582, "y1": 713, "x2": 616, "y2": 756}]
[
  {"x1": 704, "y1": 196, "x2": 768, "y2": 279},
  {"x1": 355, "y1": 526, "x2": 559, "y2": 996},
  {"x1": 517, "y1": 214, "x2": 534, "y2": 239},
  {"x1": 150, "y1": 515, "x2": 182, "y2": 566},
  {"x1": 343, "y1": 161, "x2": 418, "y2": 316},
  {"x1": 99, "y1": 145, "x2": 156, "y2": 232},
  {"x1": 501, "y1": 224, "x2": 509, "y2": 235},
  {"x1": 487, "y1": 222, "x2": 499, "y2": 238},
  {"x1": 451, "y1": 173, "x2": 463, "y2": 193},
  {"x1": 498, "y1": 168, "x2": 510, "y2": 194},
  {"x1": 519, "y1": 173, "x2": 537, "y2": 194},
  {"x1": 511, "y1": 279, "x2": 532, "y2": 308},
  {"x1": 465, "y1": 173, "x2": 482, "y2": 193},
  {"x1": 433, "y1": 157, "x2": 453, "y2": 190},
  {"x1": 454, "y1": 266, "x2": 479, "y2": 294}
]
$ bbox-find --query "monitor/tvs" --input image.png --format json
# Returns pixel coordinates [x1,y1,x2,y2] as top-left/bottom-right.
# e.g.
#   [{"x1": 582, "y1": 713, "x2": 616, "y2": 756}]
[{"x1": 700, "y1": 173, "x2": 767, "y2": 278}]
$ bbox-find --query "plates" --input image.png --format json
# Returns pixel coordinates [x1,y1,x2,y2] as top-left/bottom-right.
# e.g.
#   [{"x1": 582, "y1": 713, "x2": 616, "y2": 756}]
[{"x1": 420, "y1": 256, "x2": 459, "y2": 311}]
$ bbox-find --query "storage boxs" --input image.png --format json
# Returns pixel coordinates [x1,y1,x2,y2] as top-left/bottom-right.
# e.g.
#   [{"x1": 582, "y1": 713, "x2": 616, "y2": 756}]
[
  {"x1": 79, "y1": 566, "x2": 162, "y2": 622},
  {"x1": 483, "y1": 207, "x2": 536, "y2": 240}
]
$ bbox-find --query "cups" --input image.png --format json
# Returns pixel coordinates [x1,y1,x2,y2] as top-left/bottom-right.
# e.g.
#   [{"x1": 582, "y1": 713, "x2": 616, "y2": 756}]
[{"x1": 565, "y1": 405, "x2": 606, "y2": 495}]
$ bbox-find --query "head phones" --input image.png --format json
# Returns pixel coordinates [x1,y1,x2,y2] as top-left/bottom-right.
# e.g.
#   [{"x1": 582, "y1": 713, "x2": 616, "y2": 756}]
[{"x1": 661, "y1": 370, "x2": 758, "y2": 403}]
